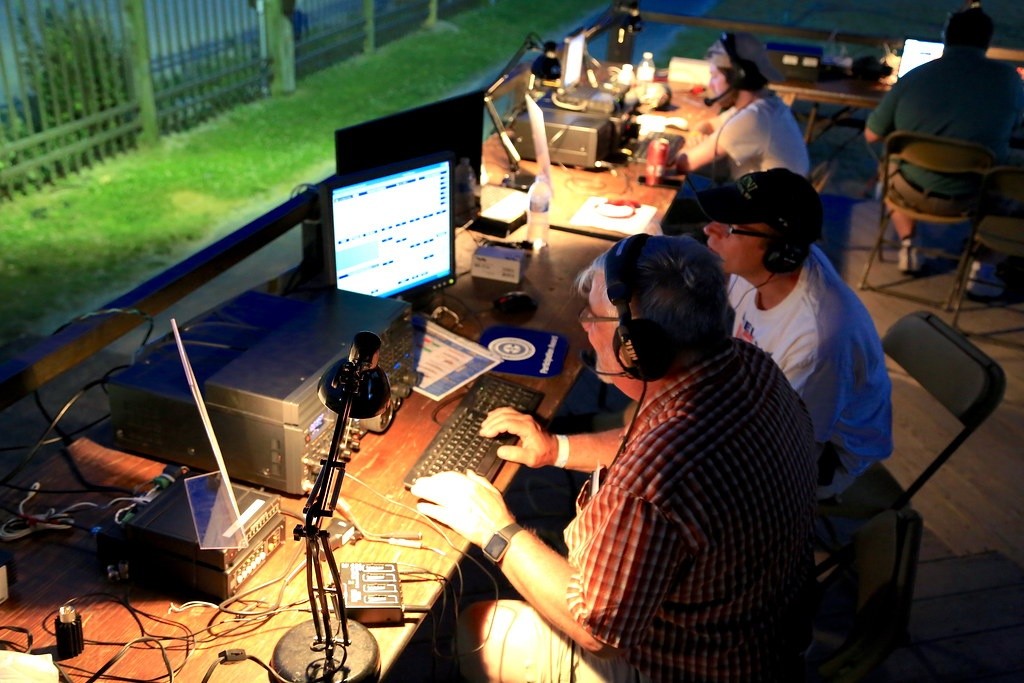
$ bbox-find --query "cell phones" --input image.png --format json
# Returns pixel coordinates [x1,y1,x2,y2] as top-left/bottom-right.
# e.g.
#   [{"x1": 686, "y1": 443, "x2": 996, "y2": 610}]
[{"x1": 638, "y1": 175, "x2": 682, "y2": 190}]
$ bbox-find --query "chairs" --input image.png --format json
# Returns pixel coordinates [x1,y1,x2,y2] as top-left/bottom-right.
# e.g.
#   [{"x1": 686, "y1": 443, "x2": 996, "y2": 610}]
[
  {"x1": 857, "y1": 128, "x2": 994, "y2": 311},
  {"x1": 771, "y1": 510, "x2": 925, "y2": 683},
  {"x1": 804, "y1": 310, "x2": 1008, "y2": 637},
  {"x1": 948, "y1": 164, "x2": 1024, "y2": 329}
]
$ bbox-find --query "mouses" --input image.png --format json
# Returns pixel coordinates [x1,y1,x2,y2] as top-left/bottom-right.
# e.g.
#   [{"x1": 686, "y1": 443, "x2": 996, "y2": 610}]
[
  {"x1": 493, "y1": 291, "x2": 538, "y2": 315},
  {"x1": 596, "y1": 203, "x2": 634, "y2": 217},
  {"x1": 664, "y1": 117, "x2": 687, "y2": 130}
]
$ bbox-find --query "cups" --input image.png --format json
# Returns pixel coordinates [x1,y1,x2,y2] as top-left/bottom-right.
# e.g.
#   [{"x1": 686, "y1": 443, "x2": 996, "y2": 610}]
[{"x1": 646, "y1": 137, "x2": 669, "y2": 187}]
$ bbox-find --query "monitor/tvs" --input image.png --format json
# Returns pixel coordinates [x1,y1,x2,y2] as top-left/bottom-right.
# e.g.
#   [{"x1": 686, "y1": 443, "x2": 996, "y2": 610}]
[
  {"x1": 898, "y1": 36, "x2": 946, "y2": 81},
  {"x1": 551, "y1": 27, "x2": 589, "y2": 109},
  {"x1": 320, "y1": 86, "x2": 486, "y2": 312}
]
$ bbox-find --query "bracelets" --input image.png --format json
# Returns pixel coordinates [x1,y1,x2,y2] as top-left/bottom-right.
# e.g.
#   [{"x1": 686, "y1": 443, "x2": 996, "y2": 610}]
[{"x1": 553, "y1": 433, "x2": 570, "y2": 469}]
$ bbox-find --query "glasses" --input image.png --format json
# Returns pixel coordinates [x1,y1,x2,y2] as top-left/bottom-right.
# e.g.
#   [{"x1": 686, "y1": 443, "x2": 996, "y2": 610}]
[
  {"x1": 577, "y1": 306, "x2": 619, "y2": 333},
  {"x1": 719, "y1": 224, "x2": 775, "y2": 240}
]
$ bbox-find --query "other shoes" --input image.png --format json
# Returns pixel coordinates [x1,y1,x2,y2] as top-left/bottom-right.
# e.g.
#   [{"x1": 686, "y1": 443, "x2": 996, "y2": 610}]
[
  {"x1": 898, "y1": 239, "x2": 925, "y2": 272},
  {"x1": 965, "y1": 262, "x2": 1005, "y2": 299}
]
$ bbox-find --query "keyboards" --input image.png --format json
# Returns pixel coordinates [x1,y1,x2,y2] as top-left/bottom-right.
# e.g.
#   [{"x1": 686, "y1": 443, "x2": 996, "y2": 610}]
[
  {"x1": 403, "y1": 372, "x2": 544, "y2": 488},
  {"x1": 632, "y1": 131, "x2": 684, "y2": 168}
]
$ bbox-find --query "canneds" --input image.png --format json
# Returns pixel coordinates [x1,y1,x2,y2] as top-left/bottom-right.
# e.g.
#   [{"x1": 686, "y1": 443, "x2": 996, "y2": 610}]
[{"x1": 645, "y1": 137, "x2": 670, "y2": 187}]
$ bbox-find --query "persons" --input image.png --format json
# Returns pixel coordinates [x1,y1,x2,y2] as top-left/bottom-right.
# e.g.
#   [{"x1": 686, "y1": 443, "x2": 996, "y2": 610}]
[
  {"x1": 693, "y1": 168, "x2": 892, "y2": 574},
  {"x1": 411, "y1": 233, "x2": 823, "y2": 683},
  {"x1": 864, "y1": 4, "x2": 1023, "y2": 299},
  {"x1": 665, "y1": 26, "x2": 811, "y2": 190}
]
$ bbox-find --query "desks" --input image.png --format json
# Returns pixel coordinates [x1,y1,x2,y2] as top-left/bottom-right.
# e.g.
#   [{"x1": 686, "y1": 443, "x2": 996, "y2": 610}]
[{"x1": 0, "y1": 44, "x2": 900, "y2": 683}]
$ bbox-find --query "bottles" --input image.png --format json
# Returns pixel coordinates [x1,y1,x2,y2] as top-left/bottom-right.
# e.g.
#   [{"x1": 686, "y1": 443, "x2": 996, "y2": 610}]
[
  {"x1": 453, "y1": 158, "x2": 476, "y2": 223},
  {"x1": 528, "y1": 176, "x2": 550, "y2": 248},
  {"x1": 637, "y1": 52, "x2": 654, "y2": 98}
]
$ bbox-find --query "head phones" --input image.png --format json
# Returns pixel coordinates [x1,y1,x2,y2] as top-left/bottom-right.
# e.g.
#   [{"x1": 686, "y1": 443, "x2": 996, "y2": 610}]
[
  {"x1": 605, "y1": 233, "x2": 677, "y2": 383},
  {"x1": 719, "y1": 32, "x2": 759, "y2": 91},
  {"x1": 763, "y1": 167, "x2": 807, "y2": 273}
]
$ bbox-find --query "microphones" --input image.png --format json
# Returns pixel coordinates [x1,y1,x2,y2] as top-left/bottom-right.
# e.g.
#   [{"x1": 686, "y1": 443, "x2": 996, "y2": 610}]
[
  {"x1": 704, "y1": 87, "x2": 732, "y2": 107},
  {"x1": 579, "y1": 350, "x2": 626, "y2": 378}
]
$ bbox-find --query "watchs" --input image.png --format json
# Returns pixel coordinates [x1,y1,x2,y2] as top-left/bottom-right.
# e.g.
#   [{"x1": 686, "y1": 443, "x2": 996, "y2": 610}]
[{"x1": 482, "y1": 521, "x2": 525, "y2": 563}]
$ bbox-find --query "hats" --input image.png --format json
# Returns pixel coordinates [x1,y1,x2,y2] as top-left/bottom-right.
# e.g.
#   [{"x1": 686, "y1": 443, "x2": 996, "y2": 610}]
[
  {"x1": 708, "y1": 31, "x2": 785, "y2": 82},
  {"x1": 693, "y1": 167, "x2": 825, "y2": 244}
]
